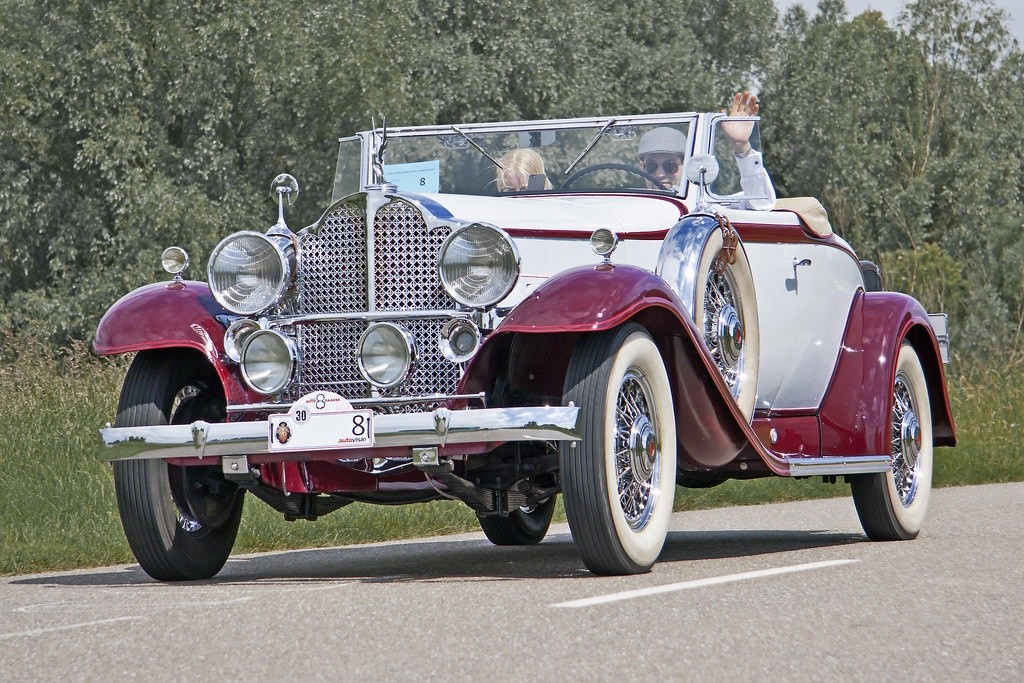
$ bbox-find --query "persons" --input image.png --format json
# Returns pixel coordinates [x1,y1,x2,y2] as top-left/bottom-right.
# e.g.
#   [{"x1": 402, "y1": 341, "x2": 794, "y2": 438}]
[
  {"x1": 496, "y1": 147, "x2": 552, "y2": 190},
  {"x1": 637, "y1": 88, "x2": 776, "y2": 212}
]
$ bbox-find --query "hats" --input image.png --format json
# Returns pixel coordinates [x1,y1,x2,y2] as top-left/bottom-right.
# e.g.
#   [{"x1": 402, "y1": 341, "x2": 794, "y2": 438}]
[{"x1": 638, "y1": 126, "x2": 686, "y2": 157}]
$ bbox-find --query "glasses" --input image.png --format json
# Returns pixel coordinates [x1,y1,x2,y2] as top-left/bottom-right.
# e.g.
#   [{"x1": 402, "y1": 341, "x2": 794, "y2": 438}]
[
  {"x1": 508, "y1": 186, "x2": 526, "y2": 191},
  {"x1": 643, "y1": 159, "x2": 678, "y2": 174}
]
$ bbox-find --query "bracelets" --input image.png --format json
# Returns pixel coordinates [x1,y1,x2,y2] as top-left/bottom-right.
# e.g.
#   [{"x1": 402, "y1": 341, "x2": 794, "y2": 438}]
[{"x1": 733, "y1": 147, "x2": 751, "y2": 158}]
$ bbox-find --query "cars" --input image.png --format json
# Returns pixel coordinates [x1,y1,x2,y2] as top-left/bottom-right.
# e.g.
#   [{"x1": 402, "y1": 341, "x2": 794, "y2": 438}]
[{"x1": 89, "y1": 109, "x2": 960, "y2": 585}]
